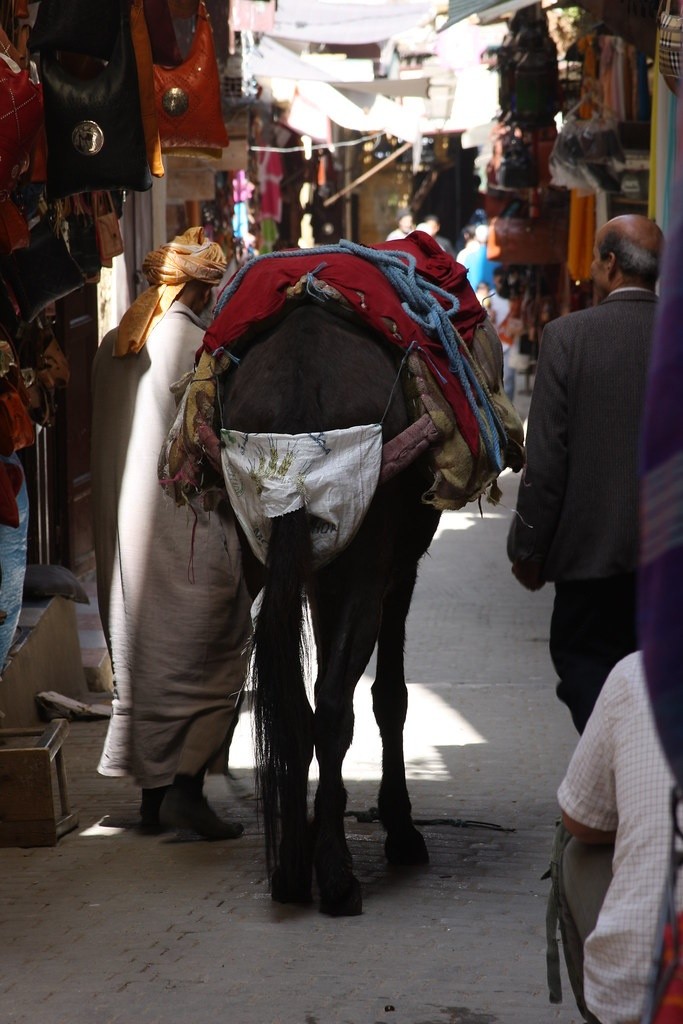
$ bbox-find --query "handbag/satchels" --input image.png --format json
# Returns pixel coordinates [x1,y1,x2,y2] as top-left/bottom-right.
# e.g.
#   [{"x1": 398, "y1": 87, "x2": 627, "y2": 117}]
[{"x1": 0, "y1": 0, "x2": 229, "y2": 528}]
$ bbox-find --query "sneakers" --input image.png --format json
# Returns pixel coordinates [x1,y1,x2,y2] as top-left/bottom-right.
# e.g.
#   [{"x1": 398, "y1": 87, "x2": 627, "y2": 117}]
[{"x1": 141, "y1": 792, "x2": 245, "y2": 838}]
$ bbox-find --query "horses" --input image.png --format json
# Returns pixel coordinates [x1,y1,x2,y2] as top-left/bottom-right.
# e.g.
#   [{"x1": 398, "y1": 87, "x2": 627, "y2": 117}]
[{"x1": 208, "y1": 293, "x2": 442, "y2": 923}]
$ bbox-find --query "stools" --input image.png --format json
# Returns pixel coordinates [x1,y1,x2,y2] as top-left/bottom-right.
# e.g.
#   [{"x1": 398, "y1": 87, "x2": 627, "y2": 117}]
[{"x1": 0, "y1": 719, "x2": 80, "y2": 849}]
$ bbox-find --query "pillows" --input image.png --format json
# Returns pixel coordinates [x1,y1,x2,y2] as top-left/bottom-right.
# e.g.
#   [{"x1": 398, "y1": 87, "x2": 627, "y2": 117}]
[{"x1": 22, "y1": 564, "x2": 92, "y2": 606}]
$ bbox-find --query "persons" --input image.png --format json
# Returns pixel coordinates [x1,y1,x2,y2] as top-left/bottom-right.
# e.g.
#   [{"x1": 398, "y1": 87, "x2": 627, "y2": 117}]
[
  {"x1": 456, "y1": 225, "x2": 518, "y2": 398},
  {"x1": 508, "y1": 214, "x2": 666, "y2": 736},
  {"x1": 550, "y1": 649, "x2": 683, "y2": 1024},
  {"x1": 94, "y1": 228, "x2": 264, "y2": 840},
  {"x1": 387, "y1": 213, "x2": 453, "y2": 256}
]
